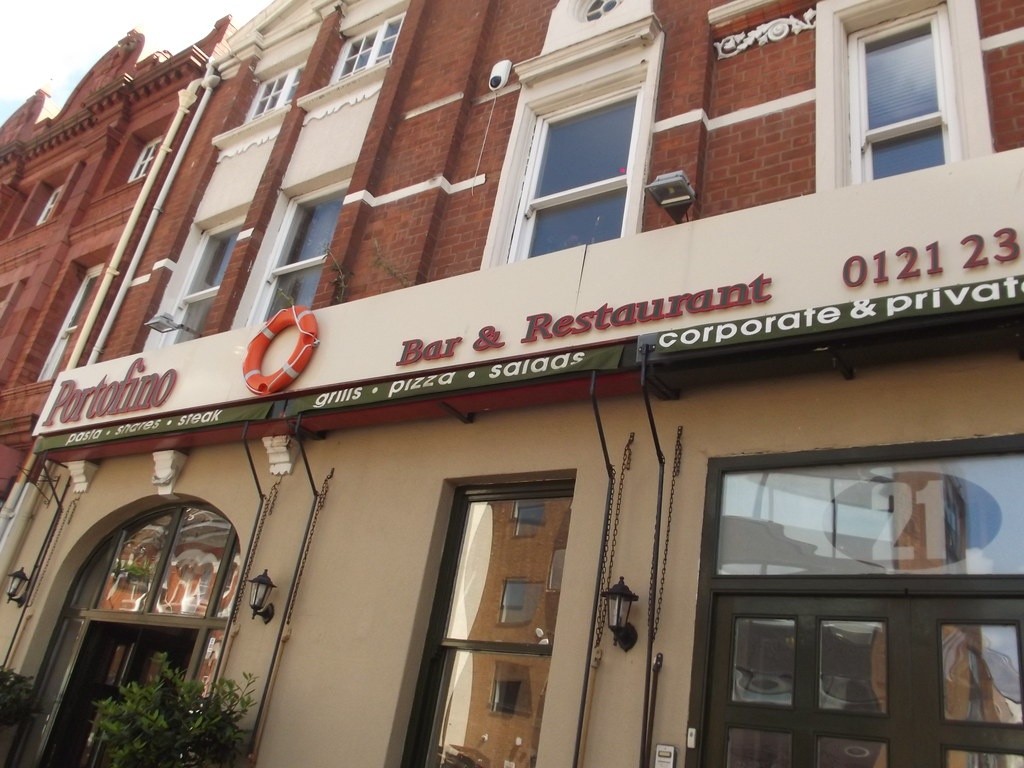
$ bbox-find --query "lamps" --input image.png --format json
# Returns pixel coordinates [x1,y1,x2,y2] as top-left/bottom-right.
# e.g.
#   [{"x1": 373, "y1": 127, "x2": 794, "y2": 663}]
[
  {"x1": 143, "y1": 311, "x2": 203, "y2": 339},
  {"x1": 645, "y1": 170, "x2": 697, "y2": 210},
  {"x1": 600, "y1": 576, "x2": 640, "y2": 652},
  {"x1": 5, "y1": 566, "x2": 30, "y2": 608},
  {"x1": 247, "y1": 569, "x2": 278, "y2": 625}
]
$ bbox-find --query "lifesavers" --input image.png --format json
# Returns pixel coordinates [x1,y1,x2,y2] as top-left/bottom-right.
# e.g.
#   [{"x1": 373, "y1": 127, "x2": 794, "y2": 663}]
[{"x1": 241, "y1": 305, "x2": 320, "y2": 396}]
